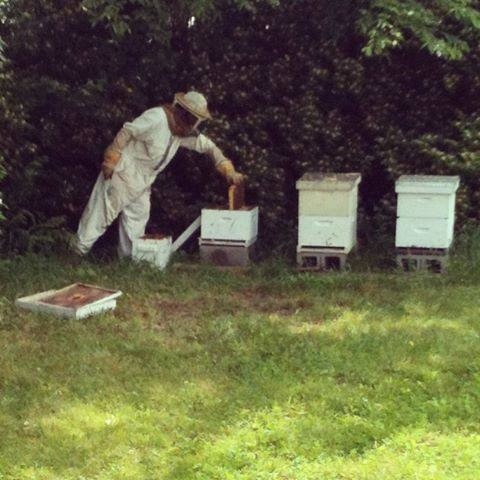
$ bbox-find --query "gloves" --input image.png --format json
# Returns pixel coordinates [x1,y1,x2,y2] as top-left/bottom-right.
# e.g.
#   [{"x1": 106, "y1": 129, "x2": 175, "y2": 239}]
[
  {"x1": 103, "y1": 127, "x2": 132, "y2": 178},
  {"x1": 217, "y1": 160, "x2": 251, "y2": 186}
]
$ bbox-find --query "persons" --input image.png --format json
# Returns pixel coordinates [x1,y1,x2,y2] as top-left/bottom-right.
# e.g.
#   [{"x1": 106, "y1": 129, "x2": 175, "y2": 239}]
[{"x1": 66, "y1": 91, "x2": 250, "y2": 265}]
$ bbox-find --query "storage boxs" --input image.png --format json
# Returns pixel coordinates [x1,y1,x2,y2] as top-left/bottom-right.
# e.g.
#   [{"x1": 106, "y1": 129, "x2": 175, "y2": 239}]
[
  {"x1": 18, "y1": 283, "x2": 122, "y2": 318},
  {"x1": 296, "y1": 171, "x2": 361, "y2": 249},
  {"x1": 395, "y1": 174, "x2": 461, "y2": 251},
  {"x1": 200, "y1": 205, "x2": 259, "y2": 248},
  {"x1": 131, "y1": 235, "x2": 174, "y2": 268}
]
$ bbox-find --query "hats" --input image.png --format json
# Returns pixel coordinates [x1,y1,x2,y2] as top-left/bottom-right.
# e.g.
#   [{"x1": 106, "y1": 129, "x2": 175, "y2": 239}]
[{"x1": 173, "y1": 90, "x2": 211, "y2": 121}]
